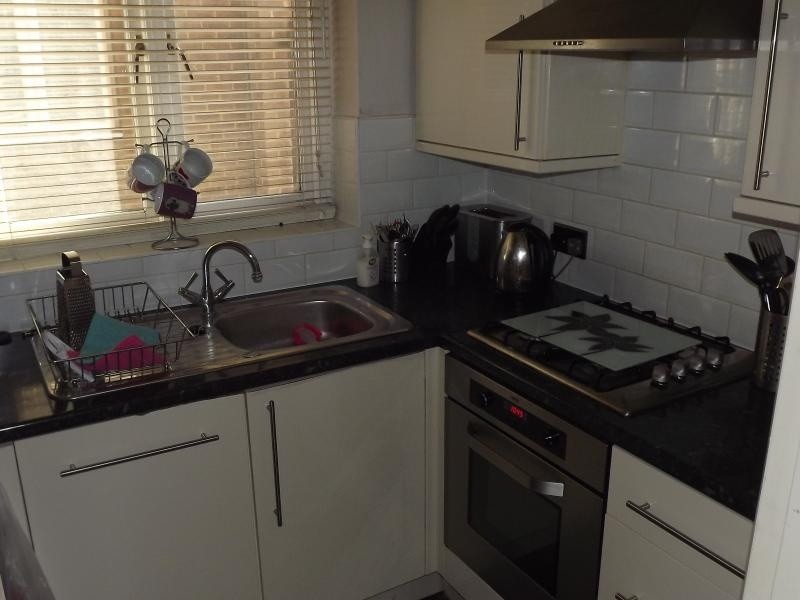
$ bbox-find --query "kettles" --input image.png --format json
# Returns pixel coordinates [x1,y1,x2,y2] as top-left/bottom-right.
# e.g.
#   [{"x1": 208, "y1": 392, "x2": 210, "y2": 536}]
[{"x1": 494, "y1": 221, "x2": 555, "y2": 303}]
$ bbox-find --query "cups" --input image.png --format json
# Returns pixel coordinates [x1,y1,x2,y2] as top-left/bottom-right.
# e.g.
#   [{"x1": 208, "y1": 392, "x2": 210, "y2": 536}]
[
  {"x1": 172, "y1": 139, "x2": 214, "y2": 190},
  {"x1": 154, "y1": 169, "x2": 197, "y2": 220},
  {"x1": 126, "y1": 142, "x2": 164, "y2": 193}
]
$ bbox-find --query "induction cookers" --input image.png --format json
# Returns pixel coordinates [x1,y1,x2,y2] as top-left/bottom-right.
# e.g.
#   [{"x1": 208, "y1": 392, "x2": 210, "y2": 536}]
[{"x1": 461, "y1": 291, "x2": 760, "y2": 425}]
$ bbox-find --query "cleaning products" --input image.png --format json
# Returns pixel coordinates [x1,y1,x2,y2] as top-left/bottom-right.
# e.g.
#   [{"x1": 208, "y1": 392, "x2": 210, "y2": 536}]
[{"x1": 355, "y1": 232, "x2": 381, "y2": 289}]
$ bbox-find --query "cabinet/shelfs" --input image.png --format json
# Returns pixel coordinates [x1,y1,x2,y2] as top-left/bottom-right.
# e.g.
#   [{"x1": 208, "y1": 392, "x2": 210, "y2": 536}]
[{"x1": 0, "y1": 349, "x2": 441, "y2": 600}]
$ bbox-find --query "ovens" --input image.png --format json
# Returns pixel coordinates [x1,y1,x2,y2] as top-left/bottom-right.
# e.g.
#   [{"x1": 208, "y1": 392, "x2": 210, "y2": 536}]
[
  {"x1": 484, "y1": 0, "x2": 771, "y2": 74},
  {"x1": 437, "y1": 349, "x2": 623, "y2": 600}
]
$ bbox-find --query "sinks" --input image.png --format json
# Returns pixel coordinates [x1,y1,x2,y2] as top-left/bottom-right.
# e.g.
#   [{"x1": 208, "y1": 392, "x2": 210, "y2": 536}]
[{"x1": 214, "y1": 298, "x2": 376, "y2": 359}]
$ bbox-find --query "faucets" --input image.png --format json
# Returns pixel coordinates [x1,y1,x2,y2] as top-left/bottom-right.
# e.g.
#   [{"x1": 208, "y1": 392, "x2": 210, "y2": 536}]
[{"x1": 199, "y1": 241, "x2": 264, "y2": 328}]
